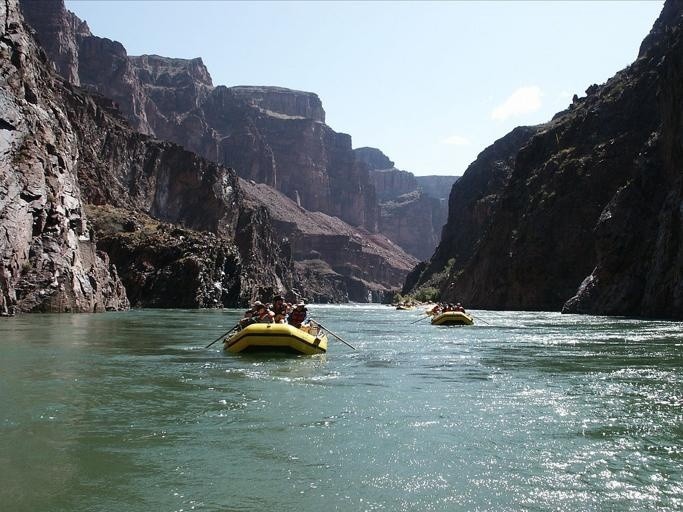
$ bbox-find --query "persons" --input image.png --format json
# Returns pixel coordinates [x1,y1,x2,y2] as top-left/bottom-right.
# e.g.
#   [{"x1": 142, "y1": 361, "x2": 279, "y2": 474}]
[
  {"x1": 430, "y1": 302, "x2": 465, "y2": 322},
  {"x1": 391, "y1": 299, "x2": 423, "y2": 308},
  {"x1": 222, "y1": 294, "x2": 323, "y2": 344}
]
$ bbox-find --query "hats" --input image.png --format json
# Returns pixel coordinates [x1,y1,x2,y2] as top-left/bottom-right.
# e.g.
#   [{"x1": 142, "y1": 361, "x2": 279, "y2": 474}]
[
  {"x1": 295, "y1": 300, "x2": 306, "y2": 306},
  {"x1": 251, "y1": 301, "x2": 261, "y2": 306}
]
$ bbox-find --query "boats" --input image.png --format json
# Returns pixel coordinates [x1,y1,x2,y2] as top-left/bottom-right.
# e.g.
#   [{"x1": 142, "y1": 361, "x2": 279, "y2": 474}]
[
  {"x1": 222, "y1": 322, "x2": 328, "y2": 355},
  {"x1": 429, "y1": 309, "x2": 475, "y2": 326},
  {"x1": 395, "y1": 304, "x2": 416, "y2": 311}
]
